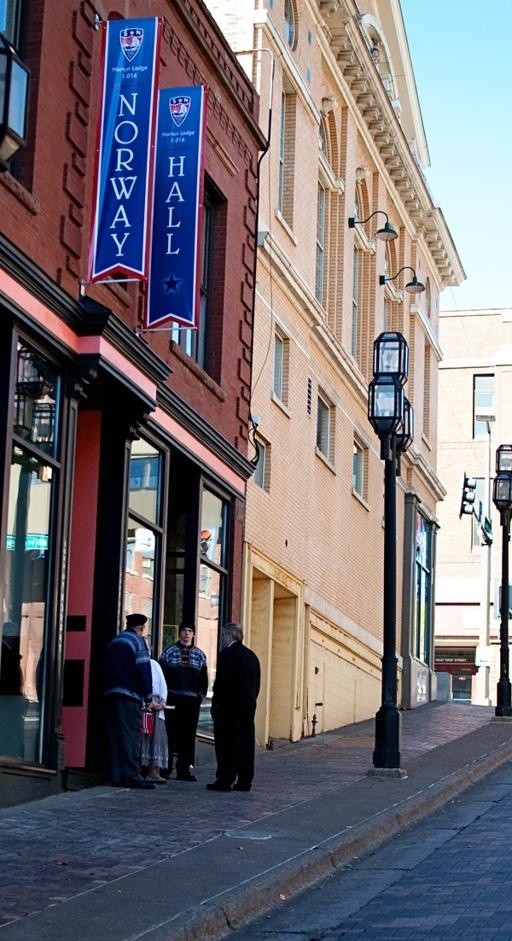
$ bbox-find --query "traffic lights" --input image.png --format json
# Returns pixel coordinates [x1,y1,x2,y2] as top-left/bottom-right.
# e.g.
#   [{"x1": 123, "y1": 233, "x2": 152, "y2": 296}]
[{"x1": 456, "y1": 472, "x2": 478, "y2": 518}]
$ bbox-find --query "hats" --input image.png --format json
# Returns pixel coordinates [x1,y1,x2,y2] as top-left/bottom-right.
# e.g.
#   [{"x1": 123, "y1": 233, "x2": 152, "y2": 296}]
[
  {"x1": 126, "y1": 614, "x2": 147, "y2": 628},
  {"x1": 179, "y1": 621, "x2": 195, "y2": 635},
  {"x1": 2, "y1": 622, "x2": 20, "y2": 637}
]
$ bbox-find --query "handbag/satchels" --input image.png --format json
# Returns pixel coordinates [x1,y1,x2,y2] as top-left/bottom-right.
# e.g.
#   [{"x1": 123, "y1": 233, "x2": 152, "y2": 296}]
[{"x1": 143, "y1": 711, "x2": 152, "y2": 735}]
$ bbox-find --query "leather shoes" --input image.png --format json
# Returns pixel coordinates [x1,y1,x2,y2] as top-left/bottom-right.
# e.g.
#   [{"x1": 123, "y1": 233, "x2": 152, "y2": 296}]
[
  {"x1": 176, "y1": 774, "x2": 197, "y2": 781},
  {"x1": 130, "y1": 768, "x2": 171, "y2": 789},
  {"x1": 233, "y1": 782, "x2": 250, "y2": 790},
  {"x1": 206, "y1": 780, "x2": 231, "y2": 792}
]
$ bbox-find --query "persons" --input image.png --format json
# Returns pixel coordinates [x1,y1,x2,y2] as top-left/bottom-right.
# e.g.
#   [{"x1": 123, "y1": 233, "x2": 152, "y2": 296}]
[
  {"x1": 106, "y1": 614, "x2": 260, "y2": 793},
  {"x1": 0, "y1": 621, "x2": 27, "y2": 758}
]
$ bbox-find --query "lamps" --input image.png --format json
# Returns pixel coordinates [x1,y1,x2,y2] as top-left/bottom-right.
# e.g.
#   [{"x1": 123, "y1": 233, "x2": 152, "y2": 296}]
[
  {"x1": 348, "y1": 211, "x2": 397, "y2": 241},
  {"x1": 380, "y1": 267, "x2": 425, "y2": 293}
]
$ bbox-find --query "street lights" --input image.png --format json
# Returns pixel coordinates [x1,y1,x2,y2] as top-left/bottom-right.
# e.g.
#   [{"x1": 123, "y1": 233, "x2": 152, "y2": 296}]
[
  {"x1": 364, "y1": 329, "x2": 413, "y2": 771},
  {"x1": 488, "y1": 444, "x2": 511, "y2": 717},
  {"x1": 1, "y1": 345, "x2": 54, "y2": 761}
]
